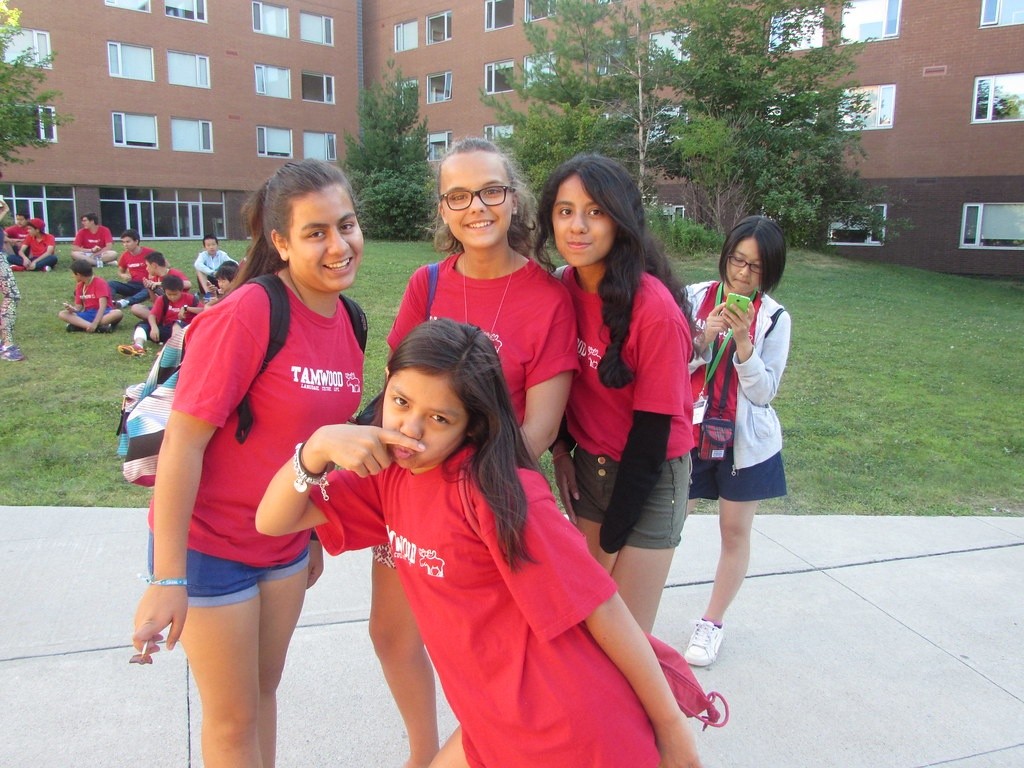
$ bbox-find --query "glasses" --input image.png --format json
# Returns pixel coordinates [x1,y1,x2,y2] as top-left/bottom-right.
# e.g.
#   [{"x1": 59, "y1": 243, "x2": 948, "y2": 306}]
[
  {"x1": 437, "y1": 186, "x2": 515, "y2": 210},
  {"x1": 727, "y1": 253, "x2": 764, "y2": 275}
]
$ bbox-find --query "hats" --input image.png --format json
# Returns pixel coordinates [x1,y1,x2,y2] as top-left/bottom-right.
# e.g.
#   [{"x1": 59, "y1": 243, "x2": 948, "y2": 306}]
[{"x1": 26, "y1": 218, "x2": 46, "y2": 232}]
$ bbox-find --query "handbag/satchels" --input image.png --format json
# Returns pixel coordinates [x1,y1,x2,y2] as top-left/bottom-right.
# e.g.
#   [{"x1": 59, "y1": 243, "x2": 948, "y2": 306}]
[
  {"x1": 647, "y1": 630, "x2": 729, "y2": 731},
  {"x1": 698, "y1": 418, "x2": 733, "y2": 461},
  {"x1": 354, "y1": 388, "x2": 388, "y2": 428}
]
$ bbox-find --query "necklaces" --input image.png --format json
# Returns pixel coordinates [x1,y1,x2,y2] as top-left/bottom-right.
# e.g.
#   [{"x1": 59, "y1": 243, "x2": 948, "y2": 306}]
[{"x1": 460, "y1": 253, "x2": 516, "y2": 334}]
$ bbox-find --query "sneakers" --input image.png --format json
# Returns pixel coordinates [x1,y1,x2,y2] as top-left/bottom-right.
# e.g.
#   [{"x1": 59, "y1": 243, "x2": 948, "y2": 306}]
[
  {"x1": 42, "y1": 266, "x2": 52, "y2": 272},
  {"x1": 118, "y1": 342, "x2": 145, "y2": 357},
  {"x1": 9, "y1": 264, "x2": 26, "y2": 272},
  {"x1": 684, "y1": 617, "x2": 725, "y2": 666},
  {"x1": 0, "y1": 343, "x2": 25, "y2": 362}
]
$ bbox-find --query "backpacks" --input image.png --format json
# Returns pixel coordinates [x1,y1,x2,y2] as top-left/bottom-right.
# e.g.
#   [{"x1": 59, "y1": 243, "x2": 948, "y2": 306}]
[{"x1": 117, "y1": 276, "x2": 370, "y2": 487}]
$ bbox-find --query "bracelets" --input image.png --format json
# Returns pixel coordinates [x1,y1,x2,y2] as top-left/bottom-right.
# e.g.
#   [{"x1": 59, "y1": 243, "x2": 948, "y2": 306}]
[
  {"x1": 139, "y1": 573, "x2": 188, "y2": 587},
  {"x1": 293, "y1": 440, "x2": 332, "y2": 501}
]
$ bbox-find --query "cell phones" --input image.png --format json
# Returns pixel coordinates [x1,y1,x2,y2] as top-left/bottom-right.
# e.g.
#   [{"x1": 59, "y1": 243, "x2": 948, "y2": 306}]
[{"x1": 723, "y1": 292, "x2": 750, "y2": 321}]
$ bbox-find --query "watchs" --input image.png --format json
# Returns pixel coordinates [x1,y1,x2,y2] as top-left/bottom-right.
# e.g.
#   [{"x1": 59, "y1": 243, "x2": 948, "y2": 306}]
[{"x1": 183, "y1": 305, "x2": 188, "y2": 311}]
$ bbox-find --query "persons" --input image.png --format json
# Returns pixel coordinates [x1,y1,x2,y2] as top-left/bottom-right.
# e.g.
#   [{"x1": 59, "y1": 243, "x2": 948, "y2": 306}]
[
  {"x1": 2, "y1": 212, "x2": 57, "y2": 271},
  {"x1": 71, "y1": 212, "x2": 118, "y2": 266},
  {"x1": 107, "y1": 228, "x2": 170, "y2": 307},
  {"x1": 130, "y1": 252, "x2": 192, "y2": 321},
  {"x1": 214, "y1": 260, "x2": 239, "y2": 293},
  {"x1": 125, "y1": 155, "x2": 371, "y2": 768},
  {"x1": 0, "y1": 172, "x2": 24, "y2": 361},
  {"x1": 192, "y1": 234, "x2": 240, "y2": 303},
  {"x1": 371, "y1": 137, "x2": 585, "y2": 768},
  {"x1": 56, "y1": 260, "x2": 123, "y2": 334},
  {"x1": 674, "y1": 215, "x2": 793, "y2": 666},
  {"x1": 526, "y1": 150, "x2": 701, "y2": 635},
  {"x1": 253, "y1": 316, "x2": 706, "y2": 768},
  {"x1": 117, "y1": 273, "x2": 204, "y2": 359}
]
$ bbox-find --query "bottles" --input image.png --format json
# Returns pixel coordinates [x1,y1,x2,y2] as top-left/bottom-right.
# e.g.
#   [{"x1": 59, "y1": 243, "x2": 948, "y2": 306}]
[{"x1": 96, "y1": 253, "x2": 103, "y2": 268}]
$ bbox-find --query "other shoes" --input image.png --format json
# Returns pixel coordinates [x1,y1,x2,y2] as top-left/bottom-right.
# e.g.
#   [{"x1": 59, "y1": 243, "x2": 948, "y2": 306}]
[
  {"x1": 104, "y1": 259, "x2": 118, "y2": 266},
  {"x1": 95, "y1": 322, "x2": 113, "y2": 333},
  {"x1": 203, "y1": 291, "x2": 212, "y2": 303},
  {"x1": 67, "y1": 324, "x2": 84, "y2": 333},
  {"x1": 112, "y1": 299, "x2": 122, "y2": 309}
]
